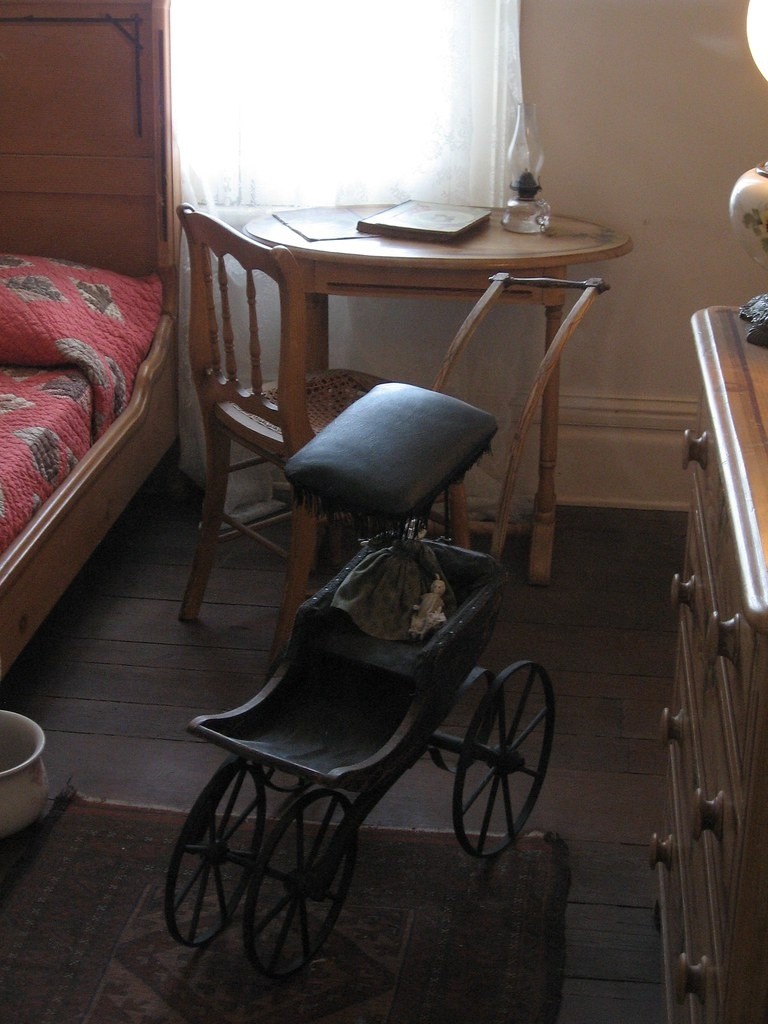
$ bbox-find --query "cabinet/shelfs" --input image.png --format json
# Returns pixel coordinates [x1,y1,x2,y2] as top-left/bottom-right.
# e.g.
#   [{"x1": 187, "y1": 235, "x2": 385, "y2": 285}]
[{"x1": 646, "y1": 306, "x2": 768, "y2": 1024}]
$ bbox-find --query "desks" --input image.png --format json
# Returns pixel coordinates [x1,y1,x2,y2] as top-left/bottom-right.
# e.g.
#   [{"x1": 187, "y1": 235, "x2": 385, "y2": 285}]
[{"x1": 245, "y1": 205, "x2": 636, "y2": 584}]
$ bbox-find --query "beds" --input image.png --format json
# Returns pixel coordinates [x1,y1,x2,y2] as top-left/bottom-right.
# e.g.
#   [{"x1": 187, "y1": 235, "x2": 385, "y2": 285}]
[{"x1": 1, "y1": 0, "x2": 180, "y2": 678}]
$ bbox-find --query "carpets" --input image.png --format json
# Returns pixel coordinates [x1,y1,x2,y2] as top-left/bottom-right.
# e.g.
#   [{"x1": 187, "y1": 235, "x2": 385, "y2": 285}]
[{"x1": 0, "y1": 784, "x2": 574, "y2": 1024}]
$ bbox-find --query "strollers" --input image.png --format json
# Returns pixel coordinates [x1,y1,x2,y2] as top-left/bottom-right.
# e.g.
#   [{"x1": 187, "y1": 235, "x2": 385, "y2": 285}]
[{"x1": 163, "y1": 272, "x2": 613, "y2": 979}]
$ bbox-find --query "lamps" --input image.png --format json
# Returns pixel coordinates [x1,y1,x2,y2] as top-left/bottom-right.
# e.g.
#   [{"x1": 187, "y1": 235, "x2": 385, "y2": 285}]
[{"x1": 728, "y1": 0, "x2": 768, "y2": 347}]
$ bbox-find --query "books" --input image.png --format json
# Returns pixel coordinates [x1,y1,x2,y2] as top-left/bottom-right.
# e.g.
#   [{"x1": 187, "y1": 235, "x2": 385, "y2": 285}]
[{"x1": 356, "y1": 199, "x2": 492, "y2": 243}]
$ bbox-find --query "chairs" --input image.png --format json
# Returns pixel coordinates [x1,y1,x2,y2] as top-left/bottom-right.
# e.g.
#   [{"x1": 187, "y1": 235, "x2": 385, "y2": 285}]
[{"x1": 175, "y1": 204, "x2": 468, "y2": 680}]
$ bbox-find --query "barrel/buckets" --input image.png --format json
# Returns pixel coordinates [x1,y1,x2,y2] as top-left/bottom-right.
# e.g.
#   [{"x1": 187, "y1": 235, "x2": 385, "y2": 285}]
[{"x1": 0, "y1": 710, "x2": 49, "y2": 838}]
